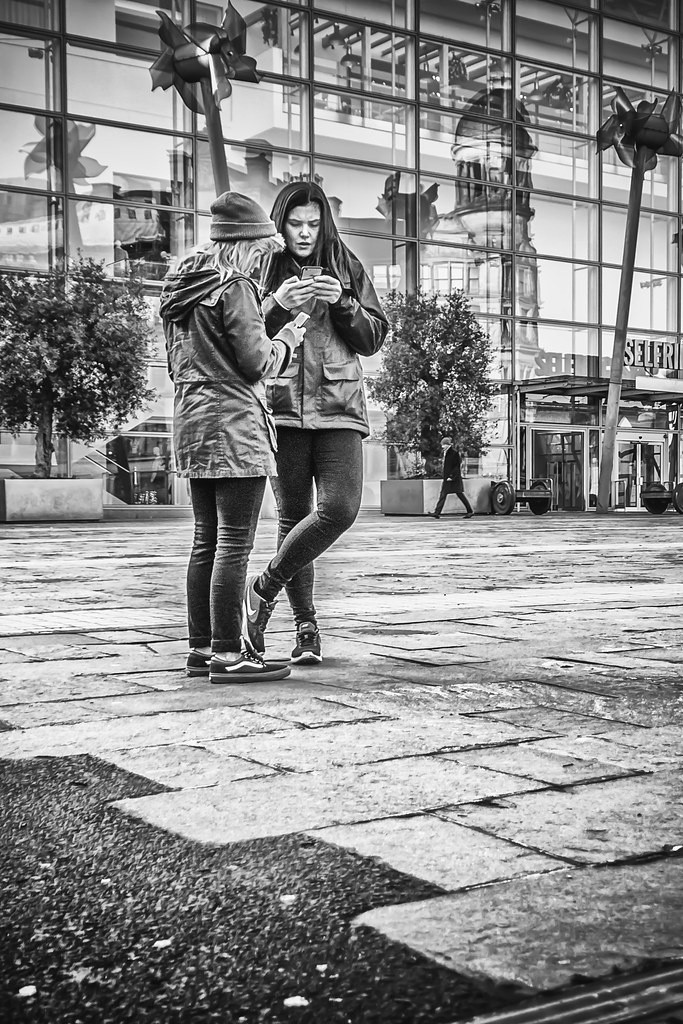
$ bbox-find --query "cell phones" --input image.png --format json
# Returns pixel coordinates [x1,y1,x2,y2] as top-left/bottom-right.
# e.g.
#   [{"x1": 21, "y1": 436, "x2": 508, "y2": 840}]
[
  {"x1": 294, "y1": 312, "x2": 310, "y2": 327},
  {"x1": 299, "y1": 266, "x2": 323, "y2": 280}
]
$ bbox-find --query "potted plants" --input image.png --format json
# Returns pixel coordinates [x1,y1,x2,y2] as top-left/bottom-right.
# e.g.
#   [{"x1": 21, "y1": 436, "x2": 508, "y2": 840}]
[
  {"x1": 0, "y1": 247, "x2": 162, "y2": 522},
  {"x1": 362, "y1": 284, "x2": 504, "y2": 514}
]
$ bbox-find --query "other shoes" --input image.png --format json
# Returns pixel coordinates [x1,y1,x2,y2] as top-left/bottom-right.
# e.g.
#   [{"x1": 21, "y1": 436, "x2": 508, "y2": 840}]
[
  {"x1": 428, "y1": 512, "x2": 439, "y2": 519},
  {"x1": 463, "y1": 511, "x2": 474, "y2": 518}
]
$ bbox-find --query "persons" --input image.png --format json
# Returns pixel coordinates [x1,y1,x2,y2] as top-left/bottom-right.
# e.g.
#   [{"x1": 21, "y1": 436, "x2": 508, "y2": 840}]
[
  {"x1": 427, "y1": 438, "x2": 476, "y2": 520},
  {"x1": 151, "y1": 446, "x2": 166, "y2": 507},
  {"x1": 242, "y1": 179, "x2": 392, "y2": 663},
  {"x1": 115, "y1": 239, "x2": 133, "y2": 279},
  {"x1": 161, "y1": 190, "x2": 310, "y2": 684}
]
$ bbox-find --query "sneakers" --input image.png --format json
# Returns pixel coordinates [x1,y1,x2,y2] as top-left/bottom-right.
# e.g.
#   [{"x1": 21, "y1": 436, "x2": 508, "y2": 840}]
[
  {"x1": 185, "y1": 650, "x2": 215, "y2": 677},
  {"x1": 241, "y1": 574, "x2": 278, "y2": 656},
  {"x1": 208, "y1": 635, "x2": 291, "y2": 684},
  {"x1": 291, "y1": 621, "x2": 323, "y2": 664}
]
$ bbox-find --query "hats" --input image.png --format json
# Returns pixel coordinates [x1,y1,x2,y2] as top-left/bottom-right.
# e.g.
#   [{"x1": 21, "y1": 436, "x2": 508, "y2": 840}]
[
  {"x1": 441, "y1": 437, "x2": 454, "y2": 445},
  {"x1": 210, "y1": 191, "x2": 277, "y2": 241}
]
